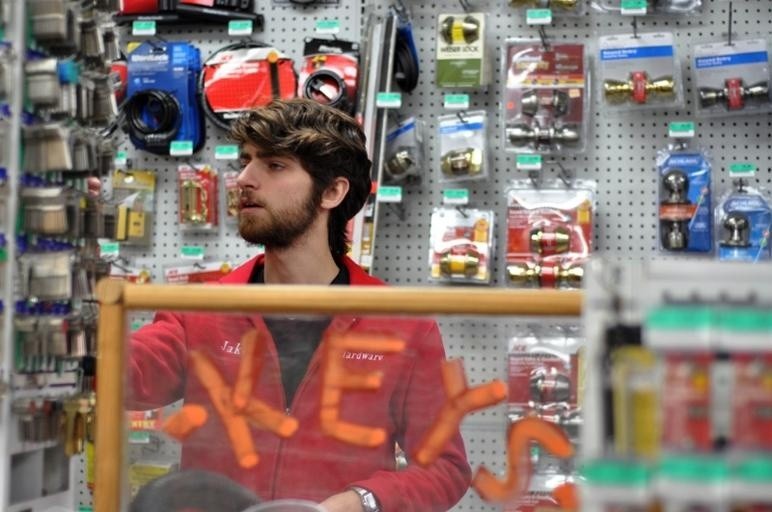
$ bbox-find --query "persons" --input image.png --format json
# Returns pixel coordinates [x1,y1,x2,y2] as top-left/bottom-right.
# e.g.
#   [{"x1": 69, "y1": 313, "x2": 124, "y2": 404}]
[{"x1": 91, "y1": 95, "x2": 474, "y2": 511}]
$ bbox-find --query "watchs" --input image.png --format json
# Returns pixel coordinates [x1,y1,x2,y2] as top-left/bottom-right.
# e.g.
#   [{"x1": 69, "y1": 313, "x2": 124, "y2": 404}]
[{"x1": 349, "y1": 485, "x2": 381, "y2": 511}]
